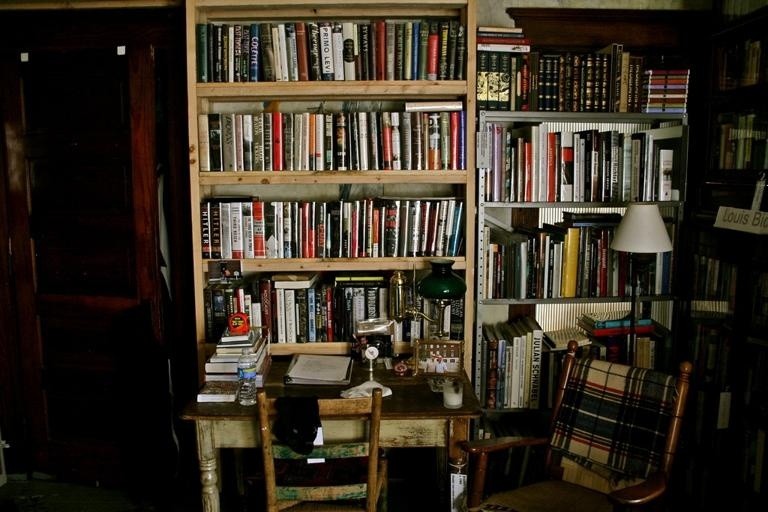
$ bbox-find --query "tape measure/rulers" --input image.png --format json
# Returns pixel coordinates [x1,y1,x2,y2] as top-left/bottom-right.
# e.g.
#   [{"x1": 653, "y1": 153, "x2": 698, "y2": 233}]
[{"x1": 228, "y1": 312, "x2": 251, "y2": 336}]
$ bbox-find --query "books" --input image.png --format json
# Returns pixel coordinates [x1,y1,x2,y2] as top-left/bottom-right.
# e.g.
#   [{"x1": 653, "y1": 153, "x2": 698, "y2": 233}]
[
  {"x1": 679, "y1": 36, "x2": 767, "y2": 436},
  {"x1": 196, "y1": 19, "x2": 468, "y2": 405},
  {"x1": 476, "y1": 25, "x2": 691, "y2": 410},
  {"x1": 741, "y1": 427, "x2": 766, "y2": 494}
]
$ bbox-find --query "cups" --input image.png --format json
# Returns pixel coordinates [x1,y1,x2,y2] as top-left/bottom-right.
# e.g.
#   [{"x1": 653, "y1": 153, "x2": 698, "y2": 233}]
[
  {"x1": 358, "y1": 344, "x2": 379, "y2": 372},
  {"x1": 443, "y1": 379, "x2": 464, "y2": 410}
]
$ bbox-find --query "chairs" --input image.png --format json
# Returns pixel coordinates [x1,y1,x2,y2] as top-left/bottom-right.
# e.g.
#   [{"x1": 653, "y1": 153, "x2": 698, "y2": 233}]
[
  {"x1": 459, "y1": 338, "x2": 692, "y2": 511},
  {"x1": 256, "y1": 388, "x2": 391, "y2": 512}
]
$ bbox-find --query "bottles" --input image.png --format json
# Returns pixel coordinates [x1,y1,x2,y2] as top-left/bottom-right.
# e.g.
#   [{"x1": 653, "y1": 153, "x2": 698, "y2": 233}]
[{"x1": 236, "y1": 347, "x2": 258, "y2": 407}]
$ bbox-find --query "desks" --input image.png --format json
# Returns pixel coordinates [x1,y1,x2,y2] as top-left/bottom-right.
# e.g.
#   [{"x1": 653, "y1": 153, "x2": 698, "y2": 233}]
[{"x1": 179, "y1": 358, "x2": 483, "y2": 512}]
